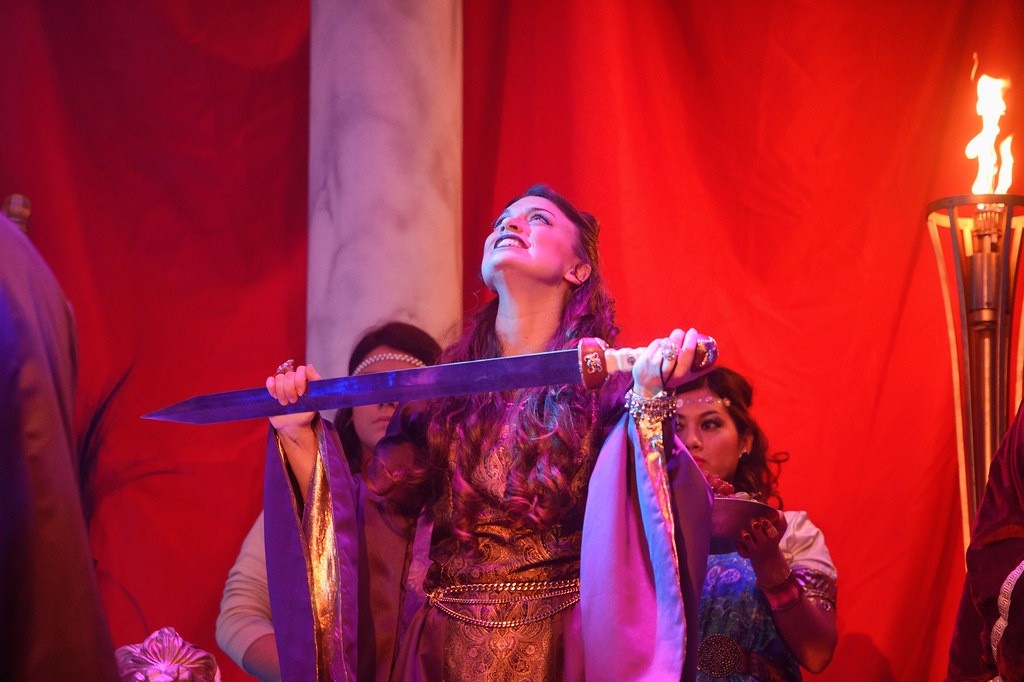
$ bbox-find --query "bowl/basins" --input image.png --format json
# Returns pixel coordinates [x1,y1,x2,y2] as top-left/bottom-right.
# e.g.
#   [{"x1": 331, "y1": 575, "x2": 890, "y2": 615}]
[{"x1": 709, "y1": 496, "x2": 779, "y2": 555}]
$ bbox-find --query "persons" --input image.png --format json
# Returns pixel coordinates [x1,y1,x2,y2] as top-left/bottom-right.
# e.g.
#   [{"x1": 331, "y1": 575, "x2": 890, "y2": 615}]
[
  {"x1": 263, "y1": 183, "x2": 708, "y2": 681},
  {"x1": 673, "y1": 362, "x2": 840, "y2": 681},
  {"x1": 214, "y1": 321, "x2": 443, "y2": 682}
]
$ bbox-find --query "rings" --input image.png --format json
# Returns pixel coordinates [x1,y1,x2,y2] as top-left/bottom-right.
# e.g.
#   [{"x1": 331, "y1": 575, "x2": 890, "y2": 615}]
[
  {"x1": 661, "y1": 339, "x2": 680, "y2": 360},
  {"x1": 275, "y1": 358, "x2": 295, "y2": 372}
]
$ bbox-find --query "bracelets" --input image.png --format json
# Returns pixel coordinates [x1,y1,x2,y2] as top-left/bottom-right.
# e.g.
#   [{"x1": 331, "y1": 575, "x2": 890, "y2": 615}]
[
  {"x1": 622, "y1": 387, "x2": 677, "y2": 421},
  {"x1": 760, "y1": 569, "x2": 804, "y2": 614}
]
